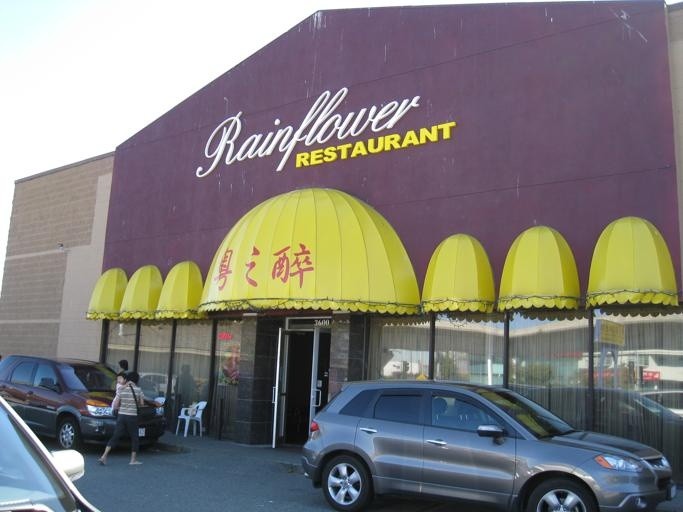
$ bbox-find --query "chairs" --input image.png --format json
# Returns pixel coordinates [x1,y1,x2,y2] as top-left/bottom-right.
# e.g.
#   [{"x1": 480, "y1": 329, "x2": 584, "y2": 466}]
[
  {"x1": 174, "y1": 397, "x2": 207, "y2": 437},
  {"x1": 431, "y1": 397, "x2": 481, "y2": 431}
]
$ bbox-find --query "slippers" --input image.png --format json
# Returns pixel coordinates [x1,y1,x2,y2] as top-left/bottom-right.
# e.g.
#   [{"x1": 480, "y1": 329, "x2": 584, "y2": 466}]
[
  {"x1": 97, "y1": 459, "x2": 107, "y2": 466},
  {"x1": 129, "y1": 461, "x2": 143, "y2": 465}
]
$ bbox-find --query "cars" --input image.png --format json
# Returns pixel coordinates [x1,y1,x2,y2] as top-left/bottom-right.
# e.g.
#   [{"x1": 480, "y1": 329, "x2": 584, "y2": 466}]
[
  {"x1": 0, "y1": 396, "x2": 101, "y2": 512},
  {"x1": 1, "y1": 352, "x2": 168, "y2": 451},
  {"x1": 140, "y1": 370, "x2": 174, "y2": 395},
  {"x1": 524, "y1": 387, "x2": 682, "y2": 469},
  {"x1": 303, "y1": 380, "x2": 678, "y2": 511}
]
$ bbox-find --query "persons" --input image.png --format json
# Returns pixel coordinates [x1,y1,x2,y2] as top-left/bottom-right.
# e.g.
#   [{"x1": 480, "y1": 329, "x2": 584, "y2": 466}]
[
  {"x1": 172, "y1": 363, "x2": 196, "y2": 429},
  {"x1": 110, "y1": 372, "x2": 129, "y2": 409},
  {"x1": 115, "y1": 359, "x2": 128, "y2": 374},
  {"x1": 96, "y1": 371, "x2": 144, "y2": 465}
]
